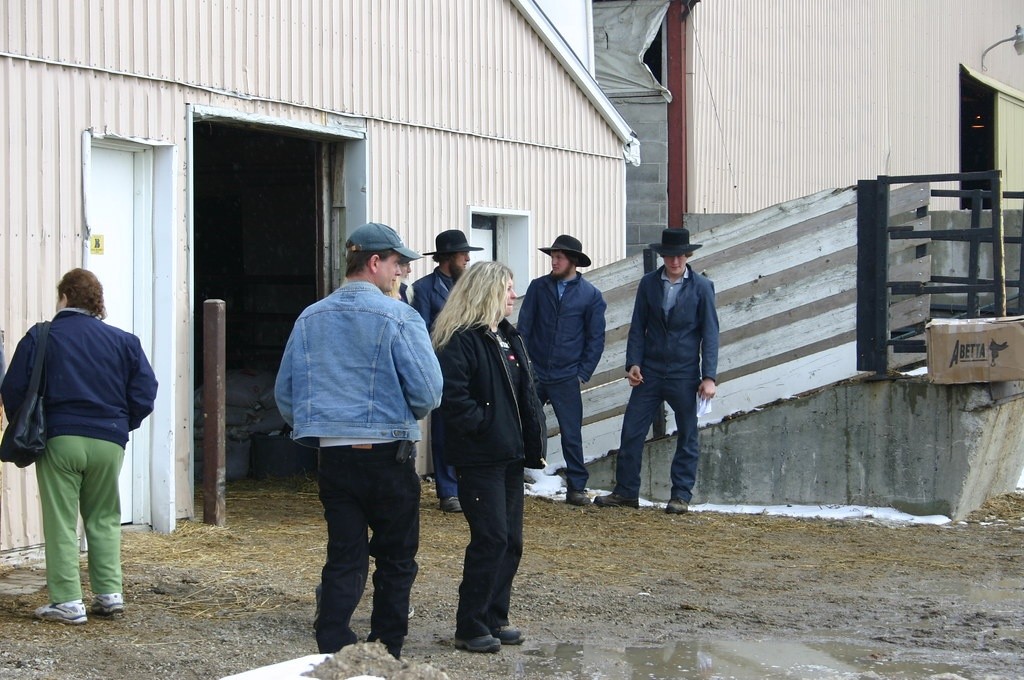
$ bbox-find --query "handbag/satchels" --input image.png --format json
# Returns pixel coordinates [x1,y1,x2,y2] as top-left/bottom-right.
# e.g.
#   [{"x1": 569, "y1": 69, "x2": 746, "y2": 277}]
[{"x1": 0, "y1": 320, "x2": 51, "y2": 469}]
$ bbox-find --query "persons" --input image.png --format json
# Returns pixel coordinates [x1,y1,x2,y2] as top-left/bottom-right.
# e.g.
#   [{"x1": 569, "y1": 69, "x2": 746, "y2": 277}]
[
  {"x1": 516, "y1": 235, "x2": 606, "y2": 504},
  {"x1": 274, "y1": 222, "x2": 443, "y2": 661},
  {"x1": 0, "y1": 268, "x2": 159, "y2": 624},
  {"x1": 431, "y1": 260, "x2": 548, "y2": 652},
  {"x1": 594, "y1": 227, "x2": 719, "y2": 515},
  {"x1": 411, "y1": 229, "x2": 485, "y2": 513}
]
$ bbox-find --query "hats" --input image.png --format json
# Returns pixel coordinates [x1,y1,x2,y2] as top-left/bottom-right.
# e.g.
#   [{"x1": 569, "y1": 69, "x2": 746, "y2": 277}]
[
  {"x1": 649, "y1": 228, "x2": 704, "y2": 257},
  {"x1": 538, "y1": 235, "x2": 591, "y2": 268},
  {"x1": 345, "y1": 222, "x2": 426, "y2": 265},
  {"x1": 423, "y1": 230, "x2": 484, "y2": 255}
]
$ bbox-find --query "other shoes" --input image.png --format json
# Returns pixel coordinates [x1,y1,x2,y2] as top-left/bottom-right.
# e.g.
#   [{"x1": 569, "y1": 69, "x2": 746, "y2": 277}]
[
  {"x1": 439, "y1": 497, "x2": 462, "y2": 511},
  {"x1": 566, "y1": 488, "x2": 591, "y2": 506}
]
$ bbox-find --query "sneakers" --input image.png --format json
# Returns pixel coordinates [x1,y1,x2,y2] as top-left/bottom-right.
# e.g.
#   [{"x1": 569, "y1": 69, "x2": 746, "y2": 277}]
[
  {"x1": 34, "y1": 603, "x2": 88, "y2": 625},
  {"x1": 90, "y1": 593, "x2": 123, "y2": 614},
  {"x1": 491, "y1": 625, "x2": 527, "y2": 644},
  {"x1": 594, "y1": 493, "x2": 639, "y2": 508},
  {"x1": 666, "y1": 497, "x2": 688, "y2": 514},
  {"x1": 455, "y1": 635, "x2": 501, "y2": 651}
]
